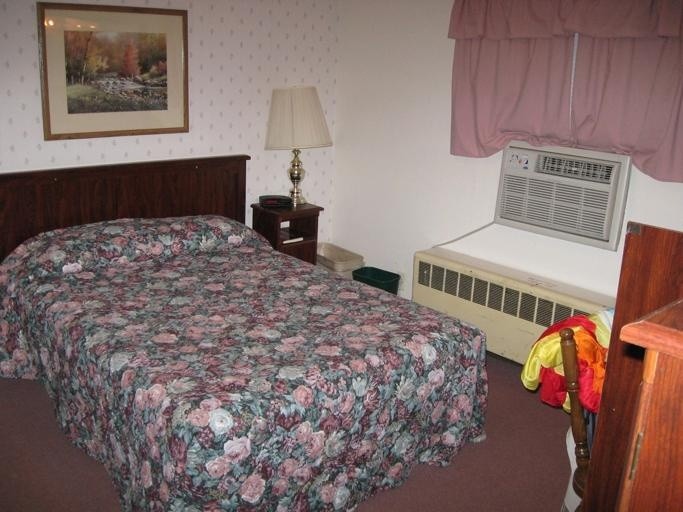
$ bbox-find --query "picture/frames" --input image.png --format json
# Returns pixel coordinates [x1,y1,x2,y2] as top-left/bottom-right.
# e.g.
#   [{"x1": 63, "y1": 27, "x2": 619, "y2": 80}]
[{"x1": 35, "y1": 1, "x2": 189, "y2": 143}]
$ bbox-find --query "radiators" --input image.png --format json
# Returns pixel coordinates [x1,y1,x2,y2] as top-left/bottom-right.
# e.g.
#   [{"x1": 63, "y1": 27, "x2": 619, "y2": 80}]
[{"x1": 411, "y1": 246, "x2": 617, "y2": 368}]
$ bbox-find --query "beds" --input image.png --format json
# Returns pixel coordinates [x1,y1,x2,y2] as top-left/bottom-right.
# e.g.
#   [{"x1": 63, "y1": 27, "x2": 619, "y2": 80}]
[{"x1": 0, "y1": 154, "x2": 489, "y2": 511}]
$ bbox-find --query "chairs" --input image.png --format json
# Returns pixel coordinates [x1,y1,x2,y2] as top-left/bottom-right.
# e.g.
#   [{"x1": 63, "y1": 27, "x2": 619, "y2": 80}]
[{"x1": 561, "y1": 308, "x2": 614, "y2": 512}]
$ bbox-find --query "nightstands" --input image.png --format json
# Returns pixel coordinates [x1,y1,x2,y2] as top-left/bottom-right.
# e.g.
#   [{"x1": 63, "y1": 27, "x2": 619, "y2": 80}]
[{"x1": 249, "y1": 201, "x2": 325, "y2": 266}]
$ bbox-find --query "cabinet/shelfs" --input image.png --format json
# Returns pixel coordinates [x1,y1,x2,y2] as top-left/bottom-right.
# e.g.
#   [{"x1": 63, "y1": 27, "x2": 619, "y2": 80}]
[{"x1": 582, "y1": 220, "x2": 683, "y2": 512}]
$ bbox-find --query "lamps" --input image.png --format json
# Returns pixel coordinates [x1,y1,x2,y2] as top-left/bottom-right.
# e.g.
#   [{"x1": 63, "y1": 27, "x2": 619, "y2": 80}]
[{"x1": 263, "y1": 85, "x2": 333, "y2": 206}]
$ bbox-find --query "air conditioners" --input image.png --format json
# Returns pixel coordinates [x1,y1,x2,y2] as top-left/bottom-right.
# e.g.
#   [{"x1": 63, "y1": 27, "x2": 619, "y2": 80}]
[{"x1": 498, "y1": 146, "x2": 621, "y2": 241}]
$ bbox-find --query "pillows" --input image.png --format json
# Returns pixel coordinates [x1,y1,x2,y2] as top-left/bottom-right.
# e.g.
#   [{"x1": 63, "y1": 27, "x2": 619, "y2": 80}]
[{"x1": 16, "y1": 212, "x2": 274, "y2": 278}]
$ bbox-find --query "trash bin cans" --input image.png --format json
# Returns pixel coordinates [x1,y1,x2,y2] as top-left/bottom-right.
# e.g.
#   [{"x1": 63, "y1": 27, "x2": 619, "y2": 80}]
[{"x1": 352, "y1": 267, "x2": 400, "y2": 296}]
